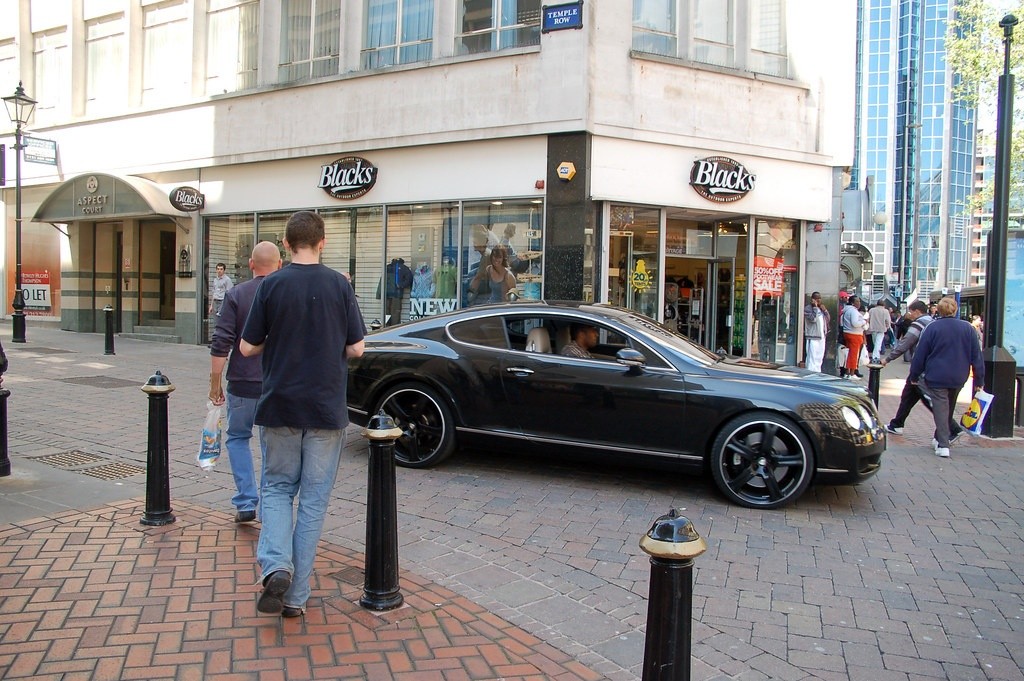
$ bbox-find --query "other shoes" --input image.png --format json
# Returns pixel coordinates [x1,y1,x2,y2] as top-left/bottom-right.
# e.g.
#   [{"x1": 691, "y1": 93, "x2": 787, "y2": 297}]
[
  {"x1": 281, "y1": 605, "x2": 302, "y2": 617},
  {"x1": 951, "y1": 426, "x2": 964, "y2": 444},
  {"x1": 235, "y1": 511, "x2": 256, "y2": 523},
  {"x1": 848, "y1": 374, "x2": 861, "y2": 381},
  {"x1": 855, "y1": 369, "x2": 863, "y2": 377},
  {"x1": 843, "y1": 374, "x2": 849, "y2": 379},
  {"x1": 257, "y1": 570, "x2": 291, "y2": 613}
]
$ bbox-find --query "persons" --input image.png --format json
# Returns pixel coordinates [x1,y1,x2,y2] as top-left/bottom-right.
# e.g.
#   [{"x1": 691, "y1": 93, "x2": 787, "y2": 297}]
[
  {"x1": 754, "y1": 291, "x2": 783, "y2": 365},
  {"x1": 209, "y1": 241, "x2": 282, "y2": 524},
  {"x1": 433, "y1": 256, "x2": 457, "y2": 301},
  {"x1": 839, "y1": 291, "x2": 870, "y2": 380},
  {"x1": 962, "y1": 312, "x2": 985, "y2": 376},
  {"x1": 803, "y1": 292, "x2": 831, "y2": 373},
  {"x1": 463, "y1": 222, "x2": 531, "y2": 305},
  {"x1": 375, "y1": 256, "x2": 413, "y2": 326},
  {"x1": 926, "y1": 303, "x2": 941, "y2": 320},
  {"x1": 561, "y1": 321, "x2": 600, "y2": 359},
  {"x1": 238, "y1": 210, "x2": 369, "y2": 619},
  {"x1": 209, "y1": 263, "x2": 234, "y2": 333},
  {"x1": 866, "y1": 299, "x2": 916, "y2": 362},
  {"x1": 879, "y1": 301, "x2": 967, "y2": 443},
  {"x1": 411, "y1": 263, "x2": 436, "y2": 320},
  {"x1": 909, "y1": 296, "x2": 986, "y2": 457}
]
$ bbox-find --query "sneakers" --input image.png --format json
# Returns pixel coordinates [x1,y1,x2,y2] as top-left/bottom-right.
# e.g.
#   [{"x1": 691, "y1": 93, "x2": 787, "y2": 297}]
[
  {"x1": 932, "y1": 438, "x2": 940, "y2": 450},
  {"x1": 935, "y1": 444, "x2": 950, "y2": 457},
  {"x1": 884, "y1": 424, "x2": 903, "y2": 434}
]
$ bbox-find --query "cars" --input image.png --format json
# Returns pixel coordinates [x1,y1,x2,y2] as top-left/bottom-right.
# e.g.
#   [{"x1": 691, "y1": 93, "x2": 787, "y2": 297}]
[{"x1": 344, "y1": 298, "x2": 889, "y2": 510}]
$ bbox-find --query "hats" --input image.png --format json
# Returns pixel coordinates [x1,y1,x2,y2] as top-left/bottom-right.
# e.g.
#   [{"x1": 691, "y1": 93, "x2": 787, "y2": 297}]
[{"x1": 840, "y1": 291, "x2": 851, "y2": 298}]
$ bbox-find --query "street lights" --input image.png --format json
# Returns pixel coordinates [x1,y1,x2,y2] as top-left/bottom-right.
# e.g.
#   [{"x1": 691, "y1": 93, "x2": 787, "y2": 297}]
[{"x1": 0, "y1": 81, "x2": 38, "y2": 342}]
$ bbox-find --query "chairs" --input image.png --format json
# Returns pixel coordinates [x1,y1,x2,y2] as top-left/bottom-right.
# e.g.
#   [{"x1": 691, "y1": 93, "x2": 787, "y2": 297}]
[
  {"x1": 525, "y1": 328, "x2": 552, "y2": 354},
  {"x1": 556, "y1": 326, "x2": 572, "y2": 355}
]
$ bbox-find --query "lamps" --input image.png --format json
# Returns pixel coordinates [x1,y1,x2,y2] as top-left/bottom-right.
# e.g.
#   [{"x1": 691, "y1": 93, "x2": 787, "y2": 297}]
[{"x1": 717, "y1": 221, "x2": 728, "y2": 234}]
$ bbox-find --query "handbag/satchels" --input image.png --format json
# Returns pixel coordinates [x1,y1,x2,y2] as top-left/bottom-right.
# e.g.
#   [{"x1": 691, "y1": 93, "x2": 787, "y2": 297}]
[
  {"x1": 960, "y1": 388, "x2": 995, "y2": 436},
  {"x1": 197, "y1": 403, "x2": 221, "y2": 471}
]
